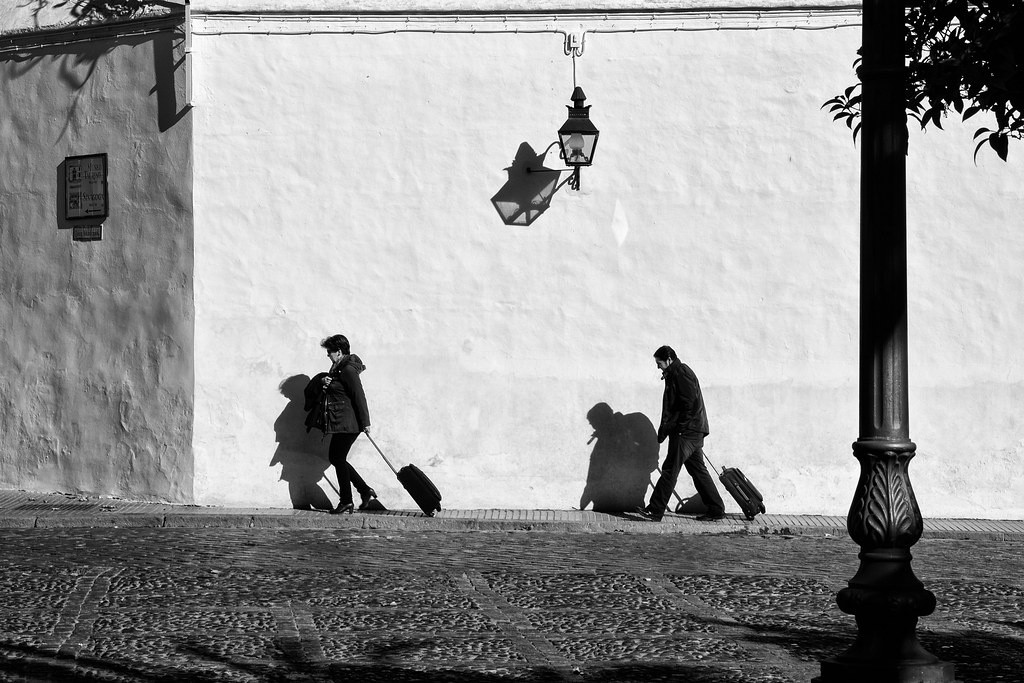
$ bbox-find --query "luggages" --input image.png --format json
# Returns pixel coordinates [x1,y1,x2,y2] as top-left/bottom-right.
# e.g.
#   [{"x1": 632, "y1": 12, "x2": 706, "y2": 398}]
[
  {"x1": 363, "y1": 427, "x2": 442, "y2": 517},
  {"x1": 702, "y1": 447, "x2": 766, "y2": 521}
]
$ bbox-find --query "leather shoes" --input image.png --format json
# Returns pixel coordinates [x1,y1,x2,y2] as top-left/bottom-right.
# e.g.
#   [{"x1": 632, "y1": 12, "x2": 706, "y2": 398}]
[
  {"x1": 635, "y1": 505, "x2": 661, "y2": 522},
  {"x1": 696, "y1": 512, "x2": 728, "y2": 521}
]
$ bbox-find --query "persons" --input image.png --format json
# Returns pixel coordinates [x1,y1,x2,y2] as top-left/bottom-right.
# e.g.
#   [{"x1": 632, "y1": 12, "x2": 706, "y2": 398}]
[
  {"x1": 320, "y1": 334, "x2": 377, "y2": 514},
  {"x1": 635, "y1": 345, "x2": 725, "y2": 522}
]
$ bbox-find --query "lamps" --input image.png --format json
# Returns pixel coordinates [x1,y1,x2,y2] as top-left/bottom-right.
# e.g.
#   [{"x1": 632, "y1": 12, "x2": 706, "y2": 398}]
[{"x1": 557, "y1": 86, "x2": 600, "y2": 193}]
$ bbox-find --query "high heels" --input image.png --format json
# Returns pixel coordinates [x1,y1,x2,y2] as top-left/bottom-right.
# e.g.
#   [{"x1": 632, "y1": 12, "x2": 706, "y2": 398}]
[
  {"x1": 329, "y1": 501, "x2": 354, "y2": 514},
  {"x1": 359, "y1": 487, "x2": 378, "y2": 510}
]
